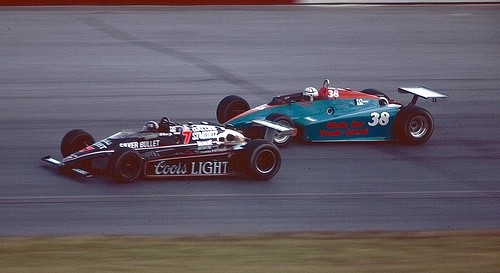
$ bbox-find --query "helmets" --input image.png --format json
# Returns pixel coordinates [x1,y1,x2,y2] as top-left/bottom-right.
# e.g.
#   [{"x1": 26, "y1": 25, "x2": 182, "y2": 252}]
[
  {"x1": 142, "y1": 121, "x2": 159, "y2": 132},
  {"x1": 303, "y1": 87, "x2": 319, "y2": 98}
]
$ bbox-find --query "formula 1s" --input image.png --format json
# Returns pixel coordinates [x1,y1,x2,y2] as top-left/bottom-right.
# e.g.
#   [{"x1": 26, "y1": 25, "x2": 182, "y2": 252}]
[
  {"x1": 216, "y1": 86, "x2": 448, "y2": 147},
  {"x1": 39, "y1": 115, "x2": 298, "y2": 183}
]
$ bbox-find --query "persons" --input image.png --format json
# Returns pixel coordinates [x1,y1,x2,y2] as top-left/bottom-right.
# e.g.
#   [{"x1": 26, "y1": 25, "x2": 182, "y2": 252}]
[
  {"x1": 142, "y1": 120, "x2": 159, "y2": 133},
  {"x1": 300, "y1": 87, "x2": 319, "y2": 101}
]
